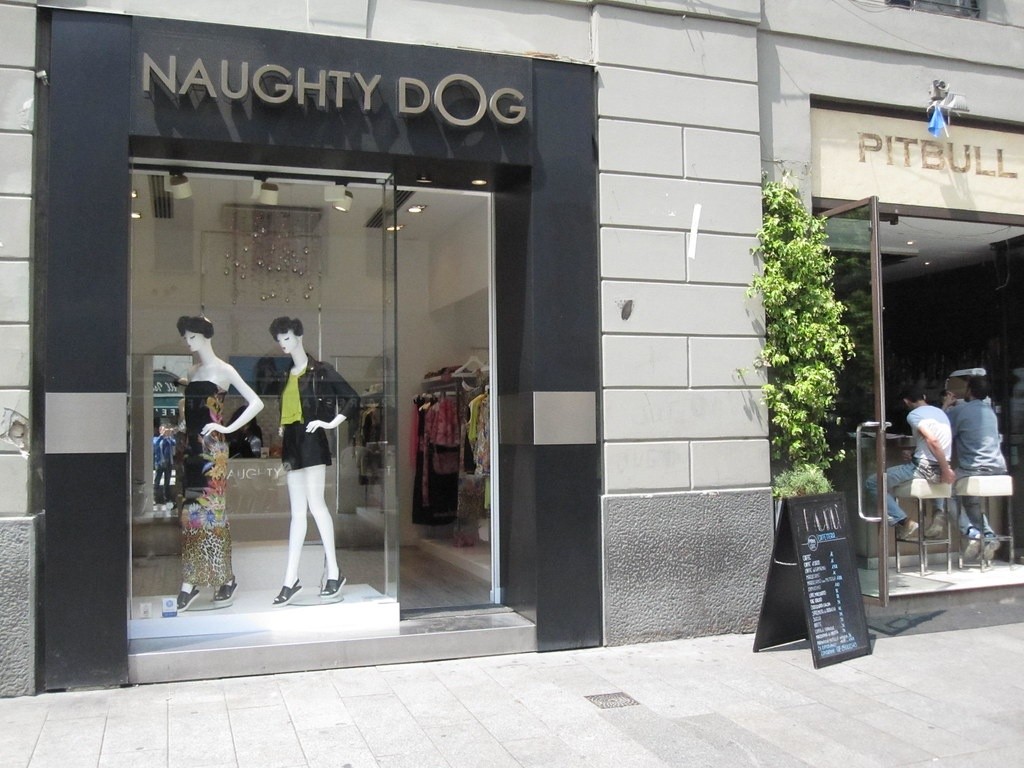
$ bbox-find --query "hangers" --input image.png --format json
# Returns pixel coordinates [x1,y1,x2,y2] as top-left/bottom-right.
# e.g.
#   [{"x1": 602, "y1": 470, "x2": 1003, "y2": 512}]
[{"x1": 357, "y1": 344, "x2": 491, "y2": 408}]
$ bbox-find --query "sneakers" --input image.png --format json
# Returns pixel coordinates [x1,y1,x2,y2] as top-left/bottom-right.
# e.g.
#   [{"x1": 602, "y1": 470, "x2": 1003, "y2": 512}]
[
  {"x1": 899, "y1": 520, "x2": 917, "y2": 539},
  {"x1": 975, "y1": 534, "x2": 1001, "y2": 564},
  {"x1": 925, "y1": 509, "x2": 947, "y2": 541},
  {"x1": 962, "y1": 529, "x2": 983, "y2": 563}
]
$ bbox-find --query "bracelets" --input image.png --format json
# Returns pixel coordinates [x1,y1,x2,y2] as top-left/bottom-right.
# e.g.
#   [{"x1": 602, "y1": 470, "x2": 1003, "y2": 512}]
[{"x1": 941, "y1": 465, "x2": 950, "y2": 472}]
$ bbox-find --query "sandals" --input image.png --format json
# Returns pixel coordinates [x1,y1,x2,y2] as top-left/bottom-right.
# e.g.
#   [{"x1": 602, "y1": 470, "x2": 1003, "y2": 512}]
[
  {"x1": 215, "y1": 576, "x2": 237, "y2": 600},
  {"x1": 177, "y1": 585, "x2": 200, "y2": 613},
  {"x1": 272, "y1": 579, "x2": 302, "y2": 607},
  {"x1": 320, "y1": 568, "x2": 346, "y2": 598}
]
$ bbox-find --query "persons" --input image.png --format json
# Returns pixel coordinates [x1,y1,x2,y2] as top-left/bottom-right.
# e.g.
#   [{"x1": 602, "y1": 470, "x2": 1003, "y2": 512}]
[
  {"x1": 866, "y1": 386, "x2": 956, "y2": 540},
  {"x1": 244, "y1": 425, "x2": 261, "y2": 458},
  {"x1": 153, "y1": 424, "x2": 176, "y2": 501},
  {"x1": 177, "y1": 316, "x2": 265, "y2": 613},
  {"x1": 270, "y1": 316, "x2": 361, "y2": 607},
  {"x1": 942, "y1": 376, "x2": 1007, "y2": 561}
]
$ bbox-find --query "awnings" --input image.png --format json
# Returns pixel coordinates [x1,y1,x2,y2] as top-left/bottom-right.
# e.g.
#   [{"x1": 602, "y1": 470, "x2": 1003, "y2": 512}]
[{"x1": 154, "y1": 397, "x2": 183, "y2": 416}]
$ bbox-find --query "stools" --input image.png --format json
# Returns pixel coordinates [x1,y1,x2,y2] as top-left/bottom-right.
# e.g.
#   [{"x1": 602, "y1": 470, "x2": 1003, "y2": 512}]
[
  {"x1": 955, "y1": 473, "x2": 1015, "y2": 567},
  {"x1": 894, "y1": 478, "x2": 952, "y2": 574}
]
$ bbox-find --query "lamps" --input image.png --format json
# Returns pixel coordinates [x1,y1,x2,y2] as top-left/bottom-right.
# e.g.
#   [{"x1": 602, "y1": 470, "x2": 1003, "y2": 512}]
[
  {"x1": 36, "y1": 70, "x2": 50, "y2": 85},
  {"x1": 260, "y1": 182, "x2": 279, "y2": 206},
  {"x1": 170, "y1": 175, "x2": 193, "y2": 199},
  {"x1": 334, "y1": 191, "x2": 353, "y2": 212},
  {"x1": 931, "y1": 79, "x2": 950, "y2": 100}
]
